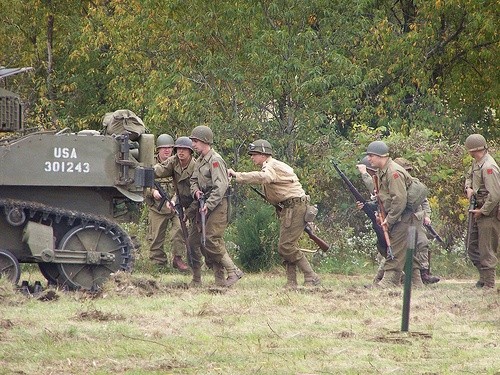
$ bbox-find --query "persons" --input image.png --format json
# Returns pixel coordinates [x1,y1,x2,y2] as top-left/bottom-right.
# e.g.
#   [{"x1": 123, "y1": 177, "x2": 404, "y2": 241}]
[
  {"x1": 227, "y1": 139, "x2": 321, "y2": 288},
  {"x1": 146, "y1": 125, "x2": 244, "y2": 292},
  {"x1": 356, "y1": 141, "x2": 441, "y2": 288},
  {"x1": 464, "y1": 134, "x2": 500, "y2": 291}
]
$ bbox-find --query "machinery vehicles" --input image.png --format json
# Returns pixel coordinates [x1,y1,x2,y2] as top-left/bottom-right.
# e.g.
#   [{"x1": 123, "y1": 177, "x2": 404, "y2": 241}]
[{"x1": 0, "y1": 88, "x2": 156, "y2": 298}]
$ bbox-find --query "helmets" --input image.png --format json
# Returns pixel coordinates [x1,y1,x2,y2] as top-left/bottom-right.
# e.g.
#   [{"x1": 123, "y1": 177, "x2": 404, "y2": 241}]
[
  {"x1": 393, "y1": 158, "x2": 413, "y2": 170},
  {"x1": 363, "y1": 140, "x2": 389, "y2": 157},
  {"x1": 464, "y1": 134, "x2": 488, "y2": 152},
  {"x1": 189, "y1": 125, "x2": 214, "y2": 144},
  {"x1": 174, "y1": 136, "x2": 194, "y2": 154},
  {"x1": 155, "y1": 134, "x2": 175, "y2": 149},
  {"x1": 247, "y1": 139, "x2": 273, "y2": 155},
  {"x1": 356, "y1": 155, "x2": 379, "y2": 172}
]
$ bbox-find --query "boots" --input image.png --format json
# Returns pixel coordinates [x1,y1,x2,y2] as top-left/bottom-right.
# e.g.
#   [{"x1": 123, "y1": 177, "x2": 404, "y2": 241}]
[
  {"x1": 364, "y1": 271, "x2": 405, "y2": 291},
  {"x1": 187, "y1": 266, "x2": 202, "y2": 290},
  {"x1": 219, "y1": 252, "x2": 244, "y2": 287},
  {"x1": 280, "y1": 264, "x2": 299, "y2": 292},
  {"x1": 469, "y1": 269, "x2": 495, "y2": 296},
  {"x1": 420, "y1": 269, "x2": 440, "y2": 285},
  {"x1": 208, "y1": 269, "x2": 227, "y2": 291},
  {"x1": 295, "y1": 255, "x2": 320, "y2": 288}
]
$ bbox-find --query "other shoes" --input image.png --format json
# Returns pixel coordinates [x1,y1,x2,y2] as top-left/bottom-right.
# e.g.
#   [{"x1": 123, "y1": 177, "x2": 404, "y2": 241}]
[{"x1": 172, "y1": 256, "x2": 189, "y2": 272}]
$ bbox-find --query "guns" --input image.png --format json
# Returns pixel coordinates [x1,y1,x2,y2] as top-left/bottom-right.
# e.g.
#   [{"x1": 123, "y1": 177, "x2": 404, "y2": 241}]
[
  {"x1": 466, "y1": 195, "x2": 475, "y2": 254},
  {"x1": 376, "y1": 205, "x2": 394, "y2": 259},
  {"x1": 178, "y1": 207, "x2": 193, "y2": 268},
  {"x1": 330, "y1": 159, "x2": 382, "y2": 231},
  {"x1": 153, "y1": 181, "x2": 178, "y2": 213},
  {"x1": 426, "y1": 223, "x2": 446, "y2": 245},
  {"x1": 198, "y1": 194, "x2": 206, "y2": 248},
  {"x1": 249, "y1": 185, "x2": 329, "y2": 253}
]
